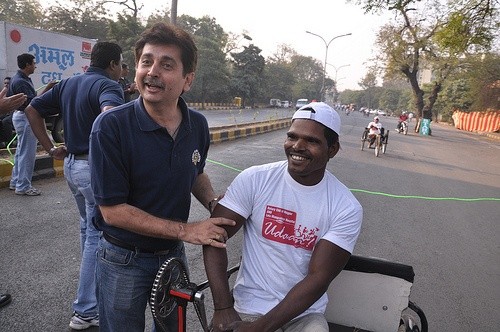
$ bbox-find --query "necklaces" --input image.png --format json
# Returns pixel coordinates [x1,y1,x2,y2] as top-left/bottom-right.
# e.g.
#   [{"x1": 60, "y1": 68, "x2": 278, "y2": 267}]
[{"x1": 172, "y1": 120, "x2": 183, "y2": 137}]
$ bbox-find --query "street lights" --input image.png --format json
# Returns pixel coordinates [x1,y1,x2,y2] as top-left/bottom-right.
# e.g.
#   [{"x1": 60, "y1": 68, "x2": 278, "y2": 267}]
[
  {"x1": 306, "y1": 30, "x2": 352, "y2": 104},
  {"x1": 327, "y1": 63, "x2": 352, "y2": 84}
]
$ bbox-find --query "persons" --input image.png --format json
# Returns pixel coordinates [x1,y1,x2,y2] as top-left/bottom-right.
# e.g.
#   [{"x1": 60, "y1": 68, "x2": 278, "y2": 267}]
[
  {"x1": 9, "y1": 54, "x2": 59, "y2": 196},
  {"x1": 0, "y1": 77, "x2": 15, "y2": 139},
  {"x1": 360, "y1": 107, "x2": 390, "y2": 116},
  {"x1": 24, "y1": 42, "x2": 125, "y2": 330},
  {"x1": 90, "y1": 23, "x2": 236, "y2": 332},
  {"x1": 119, "y1": 63, "x2": 140, "y2": 103},
  {"x1": 333, "y1": 103, "x2": 354, "y2": 115},
  {"x1": 270, "y1": 100, "x2": 294, "y2": 108},
  {"x1": 409, "y1": 112, "x2": 414, "y2": 121},
  {"x1": 0, "y1": 86, "x2": 27, "y2": 307},
  {"x1": 367, "y1": 117, "x2": 385, "y2": 149},
  {"x1": 202, "y1": 101, "x2": 364, "y2": 332},
  {"x1": 399, "y1": 113, "x2": 408, "y2": 128}
]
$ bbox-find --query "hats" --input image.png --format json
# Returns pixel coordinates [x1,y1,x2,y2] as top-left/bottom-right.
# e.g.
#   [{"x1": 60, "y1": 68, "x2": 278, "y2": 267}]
[
  {"x1": 291, "y1": 102, "x2": 342, "y2": 150},
  {"x1": 374, "y1": 116, "x2": 379, "y2": 120}
]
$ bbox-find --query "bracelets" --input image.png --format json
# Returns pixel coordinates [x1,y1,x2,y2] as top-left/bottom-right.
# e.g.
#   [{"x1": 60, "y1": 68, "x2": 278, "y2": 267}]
[
  {"x1": 209, "y1": 198, "x2": 218, "y2": 214},
  {"x1": 215, "y1": 305, "x2": 233, "y2": 311}
]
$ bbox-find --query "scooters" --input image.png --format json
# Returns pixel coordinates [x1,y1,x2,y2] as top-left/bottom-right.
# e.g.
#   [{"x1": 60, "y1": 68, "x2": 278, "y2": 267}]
[
  {"x1": 345, "y1": 108, "x2": 350, "y2": 116},
  {"x1": 395, "y1": 121, "x2": 409, "y2": 136}
]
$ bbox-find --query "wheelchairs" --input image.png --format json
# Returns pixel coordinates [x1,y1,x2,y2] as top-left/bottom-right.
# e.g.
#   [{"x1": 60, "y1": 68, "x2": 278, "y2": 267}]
[{"x1": 150, "y1": 250, "x2": 430, "y2": 332}]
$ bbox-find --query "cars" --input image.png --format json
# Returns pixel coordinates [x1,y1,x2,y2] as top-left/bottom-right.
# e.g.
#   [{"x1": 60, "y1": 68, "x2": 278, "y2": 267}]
[{"x1": 360, "y1": 107, "x2": 391, "y2": 116}]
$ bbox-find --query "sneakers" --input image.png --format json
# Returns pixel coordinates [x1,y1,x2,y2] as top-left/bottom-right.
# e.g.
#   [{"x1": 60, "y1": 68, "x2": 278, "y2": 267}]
[
  {"x1": 68, "y1": 311, "x2": 99, "y2": 329},
  {"x1": 15, "y1": 186, "x2": 40, "y2": 195},
  {"x1": 9, "y1": 185, "x2": 15, "y2": 189}
]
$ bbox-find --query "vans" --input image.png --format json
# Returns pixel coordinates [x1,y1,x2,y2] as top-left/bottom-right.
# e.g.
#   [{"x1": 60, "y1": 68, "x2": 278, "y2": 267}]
[
  {"x1": 296, "y1": 98, "x2": 309, "y2": 110},
  {"x1": 280, "y1": 101, "x2": 290, "y2": 108},
  {"x1": 269, "y1": 98, "x2": 282, "y2": 107}
]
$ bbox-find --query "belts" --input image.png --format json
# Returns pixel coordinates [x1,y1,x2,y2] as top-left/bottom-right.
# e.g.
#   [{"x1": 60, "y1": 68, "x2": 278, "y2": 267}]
[
  {"x1": 103, "y1": 232, "x2": 180, "y2": 252},
  {"x1": 67, "y1": 153, "x2": 89, "y2": 160}
]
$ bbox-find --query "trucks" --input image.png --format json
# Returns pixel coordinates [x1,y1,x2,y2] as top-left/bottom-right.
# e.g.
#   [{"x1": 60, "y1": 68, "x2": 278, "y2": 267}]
[{"x1": 0, "y1": 23, "x2": 98, "y2": 143}]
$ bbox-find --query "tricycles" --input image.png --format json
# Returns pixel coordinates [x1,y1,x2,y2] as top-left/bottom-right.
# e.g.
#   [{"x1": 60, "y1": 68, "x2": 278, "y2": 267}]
[{"x1": 360, "y1": 126, "x2": 390, "y2": 155}]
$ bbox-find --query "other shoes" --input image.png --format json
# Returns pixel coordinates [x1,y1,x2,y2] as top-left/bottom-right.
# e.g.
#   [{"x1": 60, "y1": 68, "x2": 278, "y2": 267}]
[{"x1": 0, "y1": 294, "x2": 12, "y2": 308}]
